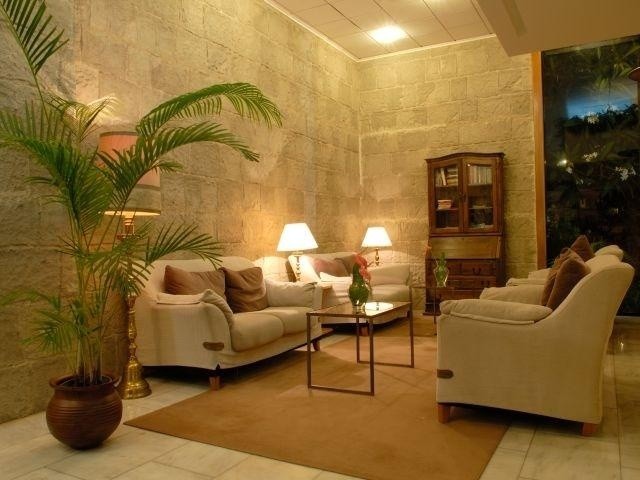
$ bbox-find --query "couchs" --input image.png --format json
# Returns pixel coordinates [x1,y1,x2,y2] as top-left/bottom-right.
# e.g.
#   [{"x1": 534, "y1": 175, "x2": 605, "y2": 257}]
[
  {"x1": 506, "y1": 245, "x2": 624, "y2": 286},
  {"x1": 117, "y1": 257, "x2": 322, "y2": 392},
  {"x1": 436, "y1": 255, "x2": 634, "y2": 436},
  {"x1": 254, "y1": 253, "x2": 410, "y2": 336}
]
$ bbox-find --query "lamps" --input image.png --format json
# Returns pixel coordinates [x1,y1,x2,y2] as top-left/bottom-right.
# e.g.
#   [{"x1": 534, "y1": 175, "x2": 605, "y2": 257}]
[
  {"x1": 361, "y1": 227, "x2": 393, "y2": 266},
  {"x1": 96, "y1": 131, "x2": 161, "y2": 400},
  {"x1": 276, "y1": 223, "x2": 319, "y2": 282}
]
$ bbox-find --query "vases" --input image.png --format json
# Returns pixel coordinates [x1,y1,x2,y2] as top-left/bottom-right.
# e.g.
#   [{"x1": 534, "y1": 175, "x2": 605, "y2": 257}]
[
  {"x1": 349, "y1": 263, "x2": 369, "y2": 307},
  {"x1": 433, "y1": 261, "x2": 449, "y2": 288}
]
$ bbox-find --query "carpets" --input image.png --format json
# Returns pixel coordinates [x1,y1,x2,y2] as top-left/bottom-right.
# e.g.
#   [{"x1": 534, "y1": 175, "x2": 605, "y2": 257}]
[{"x1": 124, "y1": 314, "x2": 509, "y2": 480}]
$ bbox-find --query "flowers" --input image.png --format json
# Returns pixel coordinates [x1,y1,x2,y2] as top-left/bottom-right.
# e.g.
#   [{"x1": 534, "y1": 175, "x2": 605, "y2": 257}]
[
  {"x1": 351, "y1": 254, "x2": 371, "y2": 284},
  {"x1": 422, "y1": 239, "x2": 445, "y2": 262}
]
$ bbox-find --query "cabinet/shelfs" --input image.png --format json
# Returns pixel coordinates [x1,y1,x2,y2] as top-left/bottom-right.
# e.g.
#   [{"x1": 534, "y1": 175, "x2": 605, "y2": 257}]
[
  {"x1": 425, "y1": 235, "x2": 503, "y2": 315},
  {"x1": 425, "y1": 152, "x2": 505, "y2": 234}
]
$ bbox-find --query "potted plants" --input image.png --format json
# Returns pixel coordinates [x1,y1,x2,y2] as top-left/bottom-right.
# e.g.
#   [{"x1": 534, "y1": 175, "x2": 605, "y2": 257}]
[{"x1": 0, "y1": 0, "x2": 284, "y2": 450}]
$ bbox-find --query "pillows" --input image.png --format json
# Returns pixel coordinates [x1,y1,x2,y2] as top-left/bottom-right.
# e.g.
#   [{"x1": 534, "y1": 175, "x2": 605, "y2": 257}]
[
  {"x1": 546, "y1": 257, "x2": 591, "y2": 311},
  {"x1": 542, "y1": 248, "x2": 582, "y2": 307},
  {"x1": 314, "y1": 257, "x2": 349, "y2": 277},
  {"x1": 571, "y1": 234, "x2": 595, "y2": 262},
  {"x1": 219, "y1": 267, "x2": 268, "y2": 312},
  {"x1": 335, "y1": 254, "x2": 354, "y2": 276},
  {"x1": 164, "y1": 265, "x2": 226, "y2": 303}
]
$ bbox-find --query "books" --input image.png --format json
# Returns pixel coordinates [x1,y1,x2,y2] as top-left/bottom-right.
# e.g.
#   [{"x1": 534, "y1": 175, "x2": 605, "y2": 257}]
[
  {"x1": 433, "y1": 167, "x2": 458, "y2": 186},
  {"x1": 466, "y1": 165, "x2": 492, "y2": 184},
  {"x1": 436, "y1": 199, "x2": 453, "y2": 210}
]
